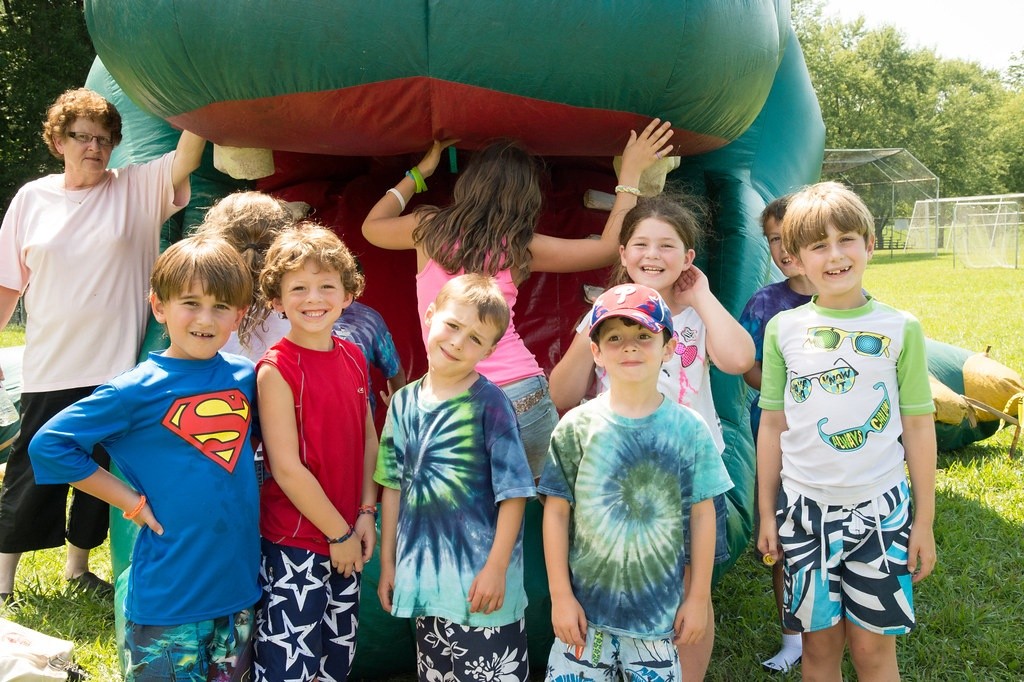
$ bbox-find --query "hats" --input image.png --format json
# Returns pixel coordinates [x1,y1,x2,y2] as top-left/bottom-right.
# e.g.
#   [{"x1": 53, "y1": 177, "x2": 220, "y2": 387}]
[{"x1": 589, "y1": 284, "x2": 673, "y2": 338}]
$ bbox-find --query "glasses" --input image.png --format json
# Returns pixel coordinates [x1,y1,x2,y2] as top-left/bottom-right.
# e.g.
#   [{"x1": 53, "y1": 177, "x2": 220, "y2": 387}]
[{"x1": 62, "y1": 132, "x2": 116, "y2": 146}]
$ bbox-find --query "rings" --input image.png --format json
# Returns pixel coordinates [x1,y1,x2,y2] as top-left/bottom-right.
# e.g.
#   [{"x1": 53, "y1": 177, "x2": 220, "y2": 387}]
[{"x1": 656, "y1": 152, "x2": 662, "y2": 159}]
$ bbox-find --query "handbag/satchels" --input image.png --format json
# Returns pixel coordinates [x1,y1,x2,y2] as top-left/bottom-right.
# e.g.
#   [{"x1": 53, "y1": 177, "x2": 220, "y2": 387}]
[{"x1": 0, "y1": 618, "x2": 89, "y2": 682}]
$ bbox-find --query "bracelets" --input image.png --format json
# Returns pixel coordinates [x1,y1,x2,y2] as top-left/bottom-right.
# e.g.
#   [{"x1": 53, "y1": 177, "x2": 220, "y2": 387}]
[
  {"x1": 406, "y1": 167, "x2": 427, "y2": 193},
  {"x1": 450, "y1": 146, "x2": 458, "y2": 172},
  {"x1": 327, "y1": 524, "x2": 354, "y2": 544},
  {"x1": 386, "y1": 188, "x2": 405, "y2": 211},
  {"x1": 615, "y1": 185, "x2": 641, "y2": 196},
  {"x1": 356, "y1": 505, "x2": 379, "y2": 520},
  {"x1": 123, "y1": 495, "x2": 147, "y2": 520}
]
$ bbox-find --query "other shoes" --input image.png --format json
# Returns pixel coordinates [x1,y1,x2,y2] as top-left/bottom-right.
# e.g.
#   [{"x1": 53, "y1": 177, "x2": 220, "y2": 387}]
[
  {"x1": 68, "y1": 571, "x2": 114, "y2": 602},
  {"x1": 763, "y1": 647, "x2": 802, "y2": 672}
]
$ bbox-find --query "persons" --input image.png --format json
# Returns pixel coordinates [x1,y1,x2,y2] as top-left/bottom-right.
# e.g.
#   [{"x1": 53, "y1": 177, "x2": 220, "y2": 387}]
[
  {"x1": 546, "y1": 181, "x2": 756, "y2": 682},
  {"x1": 0, "y1": 87, "x2": 207, "y2": 609},
  {"x1": 29, "y1": 236, "x2": 258, "y2": 682},
  {"x1": 188, "y1": 189, "x2": 406, "y2": 414},
  {"x1": 254, "y1": 219, "x2": 379, "y2": 682},
  {"x1": 371, "y1": 273, "x2": 540, "y2": 682},
  {"x1": 756, "y1": 178, "x2": 938, "y2": 682},
  {"x1": 740, "y1": 197, "x2": 819, "y2": 673},
  {"x1": 535, "y1": 284, "x2": 735, "y2": 682},
  {"x1": 361, "y1": 116, "x2": 675, "y2": 505}
]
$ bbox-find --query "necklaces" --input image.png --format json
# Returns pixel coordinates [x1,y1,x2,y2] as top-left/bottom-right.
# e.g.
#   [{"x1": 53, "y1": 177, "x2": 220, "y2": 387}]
[{"x1": 64, "y1": 170, "x2": 109, "y2": 205}]
